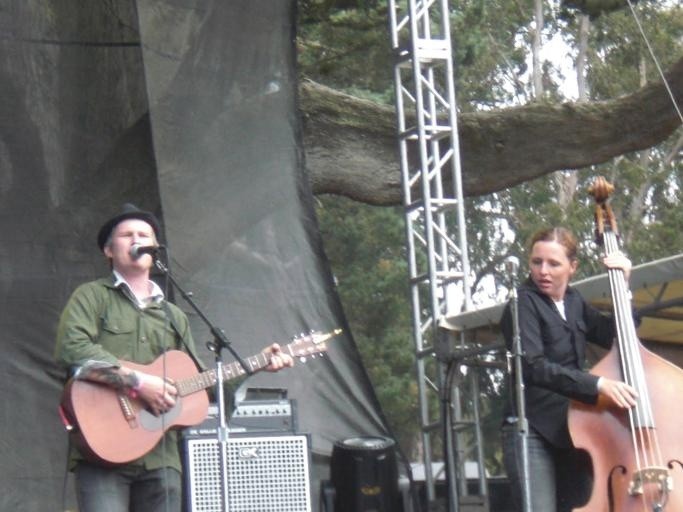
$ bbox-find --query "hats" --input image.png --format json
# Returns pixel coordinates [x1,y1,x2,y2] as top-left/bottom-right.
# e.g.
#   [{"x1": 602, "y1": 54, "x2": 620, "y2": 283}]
[{"x1": 96, "y1": 203, "x2": 161, "y2": 253}]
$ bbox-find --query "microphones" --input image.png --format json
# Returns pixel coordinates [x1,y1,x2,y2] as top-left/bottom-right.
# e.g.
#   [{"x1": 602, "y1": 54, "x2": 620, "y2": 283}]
[
  {"x1": 129, "y1": 243, "x2": 158, "y2": 256},
  {"x1": 503, "y1": 255, "x2": 521, "y2": 280}
]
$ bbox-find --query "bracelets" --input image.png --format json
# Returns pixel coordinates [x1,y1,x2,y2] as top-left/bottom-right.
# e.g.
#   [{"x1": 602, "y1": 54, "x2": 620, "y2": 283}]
[{"x1": 627, "y1": 289, "x2": 634, "y2": 301}]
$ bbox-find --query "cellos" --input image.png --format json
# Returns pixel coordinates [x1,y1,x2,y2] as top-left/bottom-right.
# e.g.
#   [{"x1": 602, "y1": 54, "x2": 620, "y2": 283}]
[{"x1": 560, "y1": 174, "x2": 682, "y2": 511}]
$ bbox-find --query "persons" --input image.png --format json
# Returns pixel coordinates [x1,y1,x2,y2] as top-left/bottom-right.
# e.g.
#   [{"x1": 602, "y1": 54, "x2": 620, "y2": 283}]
[
  {"x1": 52, "y1": 202, "x2": 295, "y2": 510},
  {"x1": 499, "y1": 226, "x2": 639, "y2": 511}
]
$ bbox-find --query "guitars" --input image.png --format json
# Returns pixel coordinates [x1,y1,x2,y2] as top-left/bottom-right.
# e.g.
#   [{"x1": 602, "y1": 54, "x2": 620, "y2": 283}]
[{"x1": 56, "y1": 327, "x2": 344, "y2": 469}]
